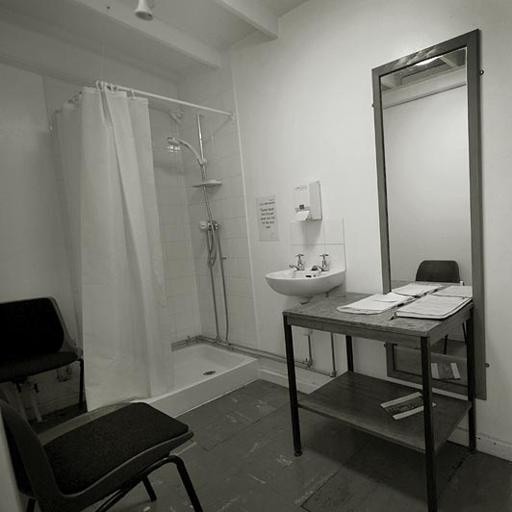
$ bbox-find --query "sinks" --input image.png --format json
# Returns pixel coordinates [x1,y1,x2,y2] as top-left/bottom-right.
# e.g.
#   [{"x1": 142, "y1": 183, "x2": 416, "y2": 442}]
[{"x1": 265, "y1": 267, "x2": 346, "y2": 296}]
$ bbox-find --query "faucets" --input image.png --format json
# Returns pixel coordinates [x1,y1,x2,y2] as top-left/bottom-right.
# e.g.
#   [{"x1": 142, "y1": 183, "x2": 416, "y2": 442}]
[
  {"x1": 312, "y1": 253, "x2": 329, "y2": 272},
  {"x1": 289, "y1": 253, "x2": 305, "y2": 270}
]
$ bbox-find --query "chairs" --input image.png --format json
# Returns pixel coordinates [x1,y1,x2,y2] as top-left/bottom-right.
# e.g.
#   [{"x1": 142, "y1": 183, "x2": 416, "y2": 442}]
[
  {"x1": 0, "y1": 298, "x2": 87, "y2": 415},
  {"x1": 1, "y1": 400, "x2": 202, "y2": 512},
  {"x1": 414, "y1": 259, "x2": 467, "y2": 355}
]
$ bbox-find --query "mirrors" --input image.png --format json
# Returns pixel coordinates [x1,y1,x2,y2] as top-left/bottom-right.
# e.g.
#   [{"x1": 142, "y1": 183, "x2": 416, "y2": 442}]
[{"x1": 372, "y1": 28, "x2": 486, "y2": 403}]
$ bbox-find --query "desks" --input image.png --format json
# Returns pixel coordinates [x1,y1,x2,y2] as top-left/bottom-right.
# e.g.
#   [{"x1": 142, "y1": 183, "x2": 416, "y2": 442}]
[{"x1": 281, "y1": 292, "x2": 476, "y2": 512}]
